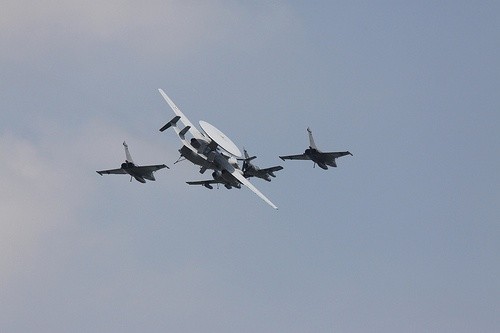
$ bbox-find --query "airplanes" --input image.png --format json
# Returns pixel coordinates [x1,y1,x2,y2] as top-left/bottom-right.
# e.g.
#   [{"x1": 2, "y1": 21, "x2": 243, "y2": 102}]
[
  {"x1": 278, "y1": 128, "x2": 354, "y2": 170},
  {"x1": 159, "y1": 89, "x2": 282, "y2": 212},
  {"x1": 96, "y1": 141, "x2": 168, "y2": 183}
]
